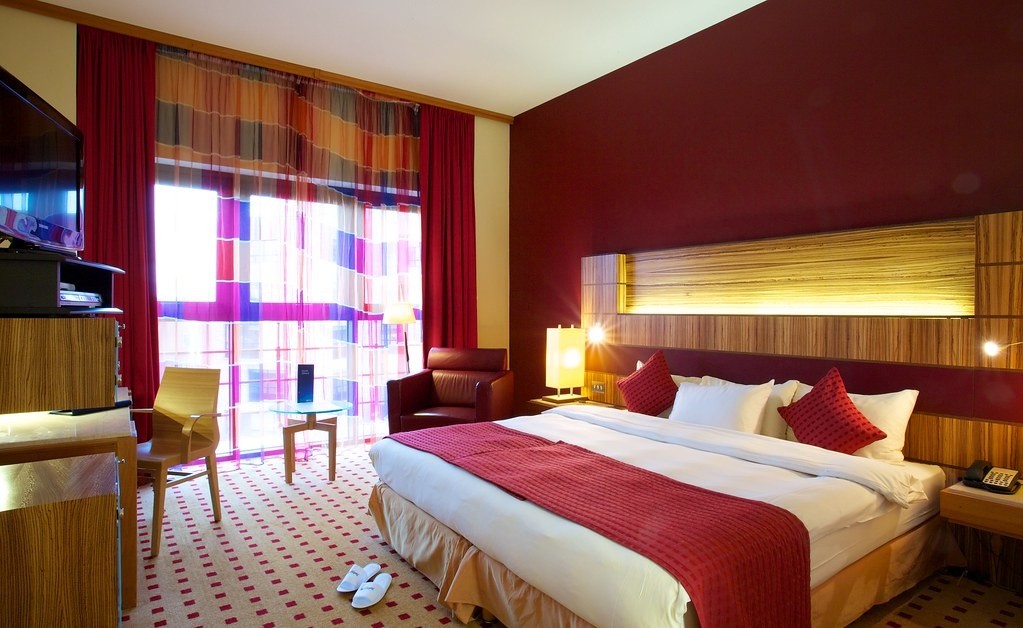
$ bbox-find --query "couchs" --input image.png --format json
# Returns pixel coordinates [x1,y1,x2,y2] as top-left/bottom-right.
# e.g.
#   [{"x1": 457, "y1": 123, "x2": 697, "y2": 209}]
[{"x1": 387, "y1": 347, "x2": 516, "y2": 436}]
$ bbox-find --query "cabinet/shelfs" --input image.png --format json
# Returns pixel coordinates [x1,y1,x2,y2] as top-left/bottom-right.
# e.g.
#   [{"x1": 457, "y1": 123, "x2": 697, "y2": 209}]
[{"x1": 0, "y1": 250, "x2": 137, "y2": 628}]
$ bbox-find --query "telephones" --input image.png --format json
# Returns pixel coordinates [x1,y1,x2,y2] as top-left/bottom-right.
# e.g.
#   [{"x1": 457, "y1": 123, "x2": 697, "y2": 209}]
[{"x1": 962, "y1": 460, "x2": 1021, "y2": 492}]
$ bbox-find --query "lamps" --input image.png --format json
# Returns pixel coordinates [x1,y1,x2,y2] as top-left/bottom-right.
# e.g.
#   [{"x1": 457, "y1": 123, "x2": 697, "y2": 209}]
[
  {"x1": 382, "y1": 302, "x2": 416, "y2": 373},
  {"x1": 542, "y1": 324, "x2": 588, "y2": 403}
]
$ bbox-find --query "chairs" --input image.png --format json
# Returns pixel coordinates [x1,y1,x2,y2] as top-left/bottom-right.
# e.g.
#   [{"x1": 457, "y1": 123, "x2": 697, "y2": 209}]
[{"x1": 130, "y1": 366, "x2": 222, "y2": 556}]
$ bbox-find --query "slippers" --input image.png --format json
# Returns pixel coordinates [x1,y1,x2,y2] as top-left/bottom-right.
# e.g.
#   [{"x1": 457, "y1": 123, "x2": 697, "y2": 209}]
[
  {"x1": 351, "y1": 573, "x2": 392, "y2": 608},
  {"x1": 337, "y1": 563, "x2": 381, "y2": 592}
]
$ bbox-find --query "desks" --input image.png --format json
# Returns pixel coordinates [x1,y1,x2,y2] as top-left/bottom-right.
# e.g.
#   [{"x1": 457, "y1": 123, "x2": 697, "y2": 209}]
[{"x1": 269, "y1": 399, "x2": 352, "y2": 483}]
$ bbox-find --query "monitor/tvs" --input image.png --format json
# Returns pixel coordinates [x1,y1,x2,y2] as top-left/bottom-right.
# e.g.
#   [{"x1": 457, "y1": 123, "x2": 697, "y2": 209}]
[{"x1": 0, "y1": 66, "x2": 85, "y2": 258}]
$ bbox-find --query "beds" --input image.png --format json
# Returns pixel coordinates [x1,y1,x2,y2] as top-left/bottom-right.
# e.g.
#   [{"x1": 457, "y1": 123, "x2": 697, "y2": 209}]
[{"x1": 367, "y1": 409, "x2": 968, "y2": 628}]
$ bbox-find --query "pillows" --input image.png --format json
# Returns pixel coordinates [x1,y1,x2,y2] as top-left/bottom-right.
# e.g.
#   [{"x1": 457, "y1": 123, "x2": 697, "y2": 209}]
[{"x1": 616, "y1": 349, "x2": 919, "y2": 463}]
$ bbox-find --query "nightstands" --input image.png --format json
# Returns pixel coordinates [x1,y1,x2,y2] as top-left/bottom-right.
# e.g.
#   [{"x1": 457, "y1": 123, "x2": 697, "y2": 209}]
[
  {"x1": 939, "y1": 480, "x2": 1023, "y2": 541},
  {"x1": 524, "y1": 398, "x2": 616, "y2": 415}
]
